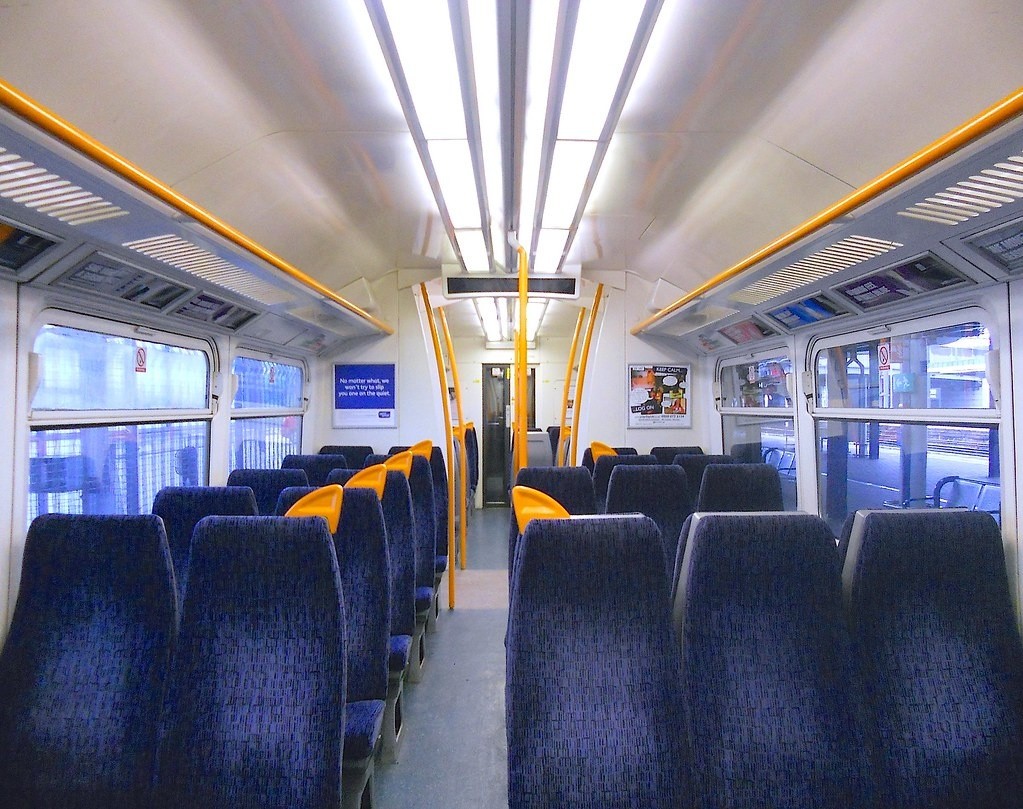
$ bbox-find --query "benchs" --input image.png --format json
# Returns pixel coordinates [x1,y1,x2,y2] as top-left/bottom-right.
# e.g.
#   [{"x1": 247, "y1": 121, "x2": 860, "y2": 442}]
[
  {"x1": 882, "y1": 475, "x2": 1002, "y2": 530},
  {"x1": 759, "y1": 444, "x2": 797, "y2": 482}
]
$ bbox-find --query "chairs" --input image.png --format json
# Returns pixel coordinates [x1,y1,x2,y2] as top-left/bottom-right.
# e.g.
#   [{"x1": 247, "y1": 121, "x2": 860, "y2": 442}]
[{"x1": 0, "y1": 427, "x2": 1023, "y2": 809}]
